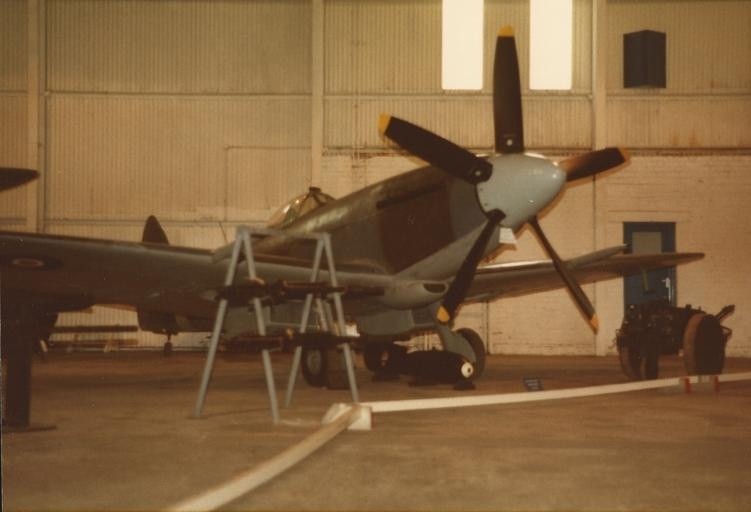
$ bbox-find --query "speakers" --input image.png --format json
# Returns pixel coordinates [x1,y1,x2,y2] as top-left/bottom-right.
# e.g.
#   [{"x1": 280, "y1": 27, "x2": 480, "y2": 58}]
[{"x1": 623, "y1": 30, "x2": 666, "y2": 88}]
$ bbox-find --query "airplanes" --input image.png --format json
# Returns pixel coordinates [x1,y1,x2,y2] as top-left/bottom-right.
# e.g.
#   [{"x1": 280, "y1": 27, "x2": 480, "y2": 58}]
[{"x1": 2, "y1": 26, "x2": 710, "y2": 397}]
